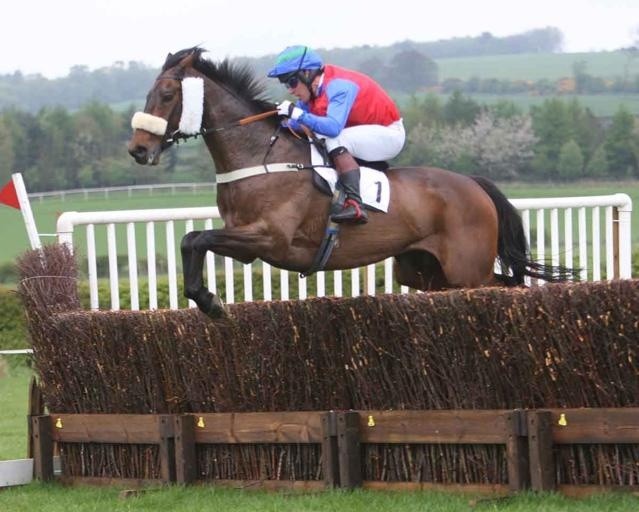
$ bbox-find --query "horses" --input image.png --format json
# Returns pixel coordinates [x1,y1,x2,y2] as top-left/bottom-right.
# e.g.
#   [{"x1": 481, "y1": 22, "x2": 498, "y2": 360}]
[{"x1": 127, "y1": 42, "x2": 593, "y2": 321}]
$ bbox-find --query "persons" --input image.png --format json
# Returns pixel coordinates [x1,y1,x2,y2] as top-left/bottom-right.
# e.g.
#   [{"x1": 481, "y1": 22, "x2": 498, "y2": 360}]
[{"x1": 266, "y1": 45, "x2": 406, "y2": 225}]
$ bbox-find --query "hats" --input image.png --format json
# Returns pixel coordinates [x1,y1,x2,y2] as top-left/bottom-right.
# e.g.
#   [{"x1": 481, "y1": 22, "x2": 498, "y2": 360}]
[{"x1": 267, "y1": 46, "x2": 322, "y2": 79}]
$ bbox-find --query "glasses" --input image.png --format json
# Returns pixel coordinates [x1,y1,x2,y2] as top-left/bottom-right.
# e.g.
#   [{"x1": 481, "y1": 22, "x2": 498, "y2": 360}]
[{"x1": 286, "y1": 77, "x2": 299, "y2": 89}]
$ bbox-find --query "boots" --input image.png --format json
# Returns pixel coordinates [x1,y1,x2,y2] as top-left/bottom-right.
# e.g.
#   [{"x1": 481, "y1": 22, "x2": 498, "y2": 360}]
[{"x1": 331, "y1": 170, "x2": 369, "y2": 225}]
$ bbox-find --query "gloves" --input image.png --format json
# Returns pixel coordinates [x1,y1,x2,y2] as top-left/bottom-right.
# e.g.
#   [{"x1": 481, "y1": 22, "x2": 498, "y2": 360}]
[{"x1": 276, "y1": 100, "x2": 304, "y2": 122}]
